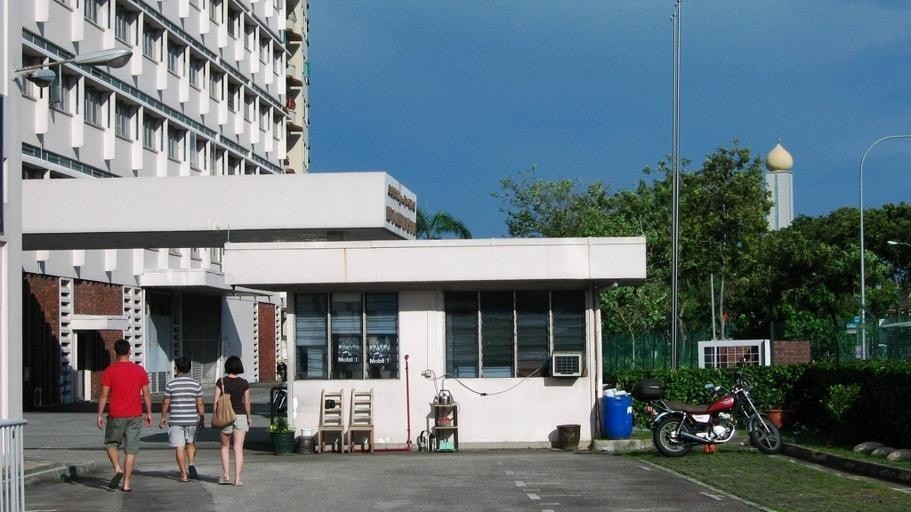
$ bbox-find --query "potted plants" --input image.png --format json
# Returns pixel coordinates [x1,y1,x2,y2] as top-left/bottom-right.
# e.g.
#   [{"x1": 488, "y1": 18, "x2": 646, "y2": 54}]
[
  {"x1": 268, "y1": 416, "x2": 294, "y2": 456},
  {"x1": 763, "y1": 388, "x2": 792, "y2": 431}
]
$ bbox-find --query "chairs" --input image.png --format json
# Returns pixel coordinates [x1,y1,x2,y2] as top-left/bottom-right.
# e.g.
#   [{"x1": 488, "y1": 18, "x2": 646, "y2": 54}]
[
  {"x1": 318, "y1": 389, "x2": 344, "y2": 453},
  {"x1": 348, "y1": 389, "x2": 374, "y2": 454}
]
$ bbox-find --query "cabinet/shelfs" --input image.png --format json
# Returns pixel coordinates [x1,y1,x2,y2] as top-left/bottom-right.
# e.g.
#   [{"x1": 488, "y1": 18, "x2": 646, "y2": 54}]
[{"x1": 435, "y1": 404, "x2": 458, "y2": 453}]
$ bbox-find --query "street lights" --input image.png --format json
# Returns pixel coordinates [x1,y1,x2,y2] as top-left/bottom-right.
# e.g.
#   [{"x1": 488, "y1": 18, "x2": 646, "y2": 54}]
[{"x1": 887, "y1": 240, "x2": 910, "y2": 248}]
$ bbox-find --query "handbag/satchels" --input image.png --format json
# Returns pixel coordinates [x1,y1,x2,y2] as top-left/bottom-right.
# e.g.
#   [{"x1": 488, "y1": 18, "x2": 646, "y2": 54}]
[{"x1": 212, "y1": 394, "x2": 237, "y2": 428}]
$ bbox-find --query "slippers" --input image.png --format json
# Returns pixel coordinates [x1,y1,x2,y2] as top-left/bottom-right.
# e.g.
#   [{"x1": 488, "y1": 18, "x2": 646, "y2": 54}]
[
  {"x1": 218, "y1": 480, "x2": 231, "y2": 483},
  {"x1": 179, "y1": 472, "x2": 188, "y2": 481},
  {"x1": 109, "y1": 472, "x2": 122, "y2": 489},
  {"x1": 188, "y1": 464, "x2": 196, "y2": 478}
]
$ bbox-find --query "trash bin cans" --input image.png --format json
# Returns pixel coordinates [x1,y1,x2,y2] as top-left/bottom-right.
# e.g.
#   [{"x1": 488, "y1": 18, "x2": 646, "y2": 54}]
[{"x1": 601, "y1": 394, "x2": 633, "y2": 440}]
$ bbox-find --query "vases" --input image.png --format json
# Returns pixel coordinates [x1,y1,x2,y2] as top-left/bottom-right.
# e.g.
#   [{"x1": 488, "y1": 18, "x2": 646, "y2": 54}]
[{"x1": 557, "y1": 424, "x2": 580, "y2": 447}]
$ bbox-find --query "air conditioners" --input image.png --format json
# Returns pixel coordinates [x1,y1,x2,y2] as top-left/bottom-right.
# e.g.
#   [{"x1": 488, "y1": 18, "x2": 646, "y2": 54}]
[{"x1": 552, "y1": 352, "x2": 583, "y2": 376}]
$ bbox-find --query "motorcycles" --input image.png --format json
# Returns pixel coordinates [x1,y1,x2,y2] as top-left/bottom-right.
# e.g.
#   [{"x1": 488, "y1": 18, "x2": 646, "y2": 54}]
[
  {"x1": 633, "y1": 354, "x2": 783, "y2": 456},
  {"x1": 268, "y1": 370, "x2": 287, "y2": 413}
]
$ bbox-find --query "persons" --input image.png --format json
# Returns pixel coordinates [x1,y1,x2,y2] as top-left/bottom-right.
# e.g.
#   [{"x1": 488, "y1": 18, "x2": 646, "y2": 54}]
[
  {"x1": 212, "y1": 356, "x2": 251, "y2": 486},
  {"x1": 159, "y1": 356, "x2": 205, "y2": 481},
  {"x1": 97, "y1": 339, "x2": 152, "y2": 491}
]
